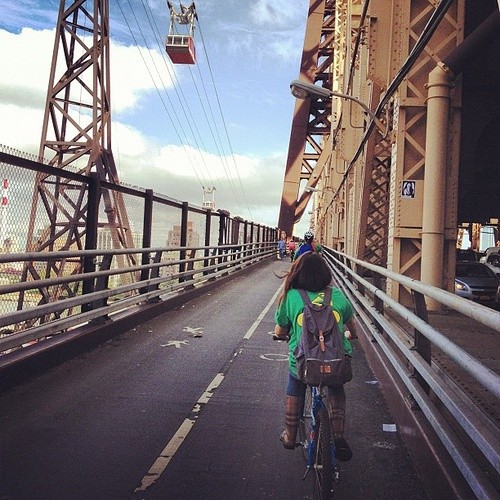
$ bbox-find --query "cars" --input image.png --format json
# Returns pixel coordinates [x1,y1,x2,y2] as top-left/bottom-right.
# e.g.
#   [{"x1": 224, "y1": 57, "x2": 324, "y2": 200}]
[
  {"x1": 456, "y1": 246, "x2": 500, "y2": 281},
  {"x1": 454, "y1": 261, "x2": 500, "y2": 305}
]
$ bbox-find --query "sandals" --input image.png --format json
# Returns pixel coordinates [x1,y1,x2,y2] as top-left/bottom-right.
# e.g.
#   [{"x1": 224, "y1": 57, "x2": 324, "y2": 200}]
[
  {"x1": 279, "y1": 432, "x2": 295, "y2": 449},
  {"x1": 334, "y1": 437, "x2": 352, "y2": 462}
]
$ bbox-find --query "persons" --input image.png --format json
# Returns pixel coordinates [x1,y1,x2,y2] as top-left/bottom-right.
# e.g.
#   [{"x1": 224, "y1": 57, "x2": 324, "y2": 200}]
[
  {"x1": 287, "y1": 240, "x2": 295, "y2": 257},
  {"x1": 293, "y1": 232, "x2": 321, "y2": 262},
  {"x1": 404, "y1": 183, "x2": 414, "y2": 195},
  {"x1": 299, "y1": 240, "x2": 319, "y2": 247},
  {"x1": 278, "y1": 238, "x2": 285, "y2": 260},
  {"x1": 273, "y1": 250, "x2": 356, "y2": 462}
]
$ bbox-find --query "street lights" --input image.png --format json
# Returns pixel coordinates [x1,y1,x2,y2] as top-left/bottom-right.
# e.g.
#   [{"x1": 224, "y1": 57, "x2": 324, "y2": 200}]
[
  {"x1": 288, "y1": 79, "x2": 397, "y2": 146},
  {"x1": 304, "y1": 185, "x2": 338, "y2": 204}
]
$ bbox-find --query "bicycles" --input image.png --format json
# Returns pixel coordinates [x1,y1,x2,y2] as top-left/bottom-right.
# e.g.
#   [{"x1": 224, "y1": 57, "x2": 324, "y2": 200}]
[{"x1": 272, "y1": 333, "x2": 359, "y2": 500}]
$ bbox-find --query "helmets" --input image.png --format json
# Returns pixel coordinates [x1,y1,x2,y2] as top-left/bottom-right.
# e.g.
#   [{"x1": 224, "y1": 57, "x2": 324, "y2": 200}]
[{"x1": 304, "y1": 231, "x2": 314, "y2": 243}]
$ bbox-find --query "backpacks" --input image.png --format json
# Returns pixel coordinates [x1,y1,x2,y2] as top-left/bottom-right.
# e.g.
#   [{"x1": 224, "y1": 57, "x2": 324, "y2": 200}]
[{"x1": 293, "y1": 287, "x2": 353, "y2": 386}]
